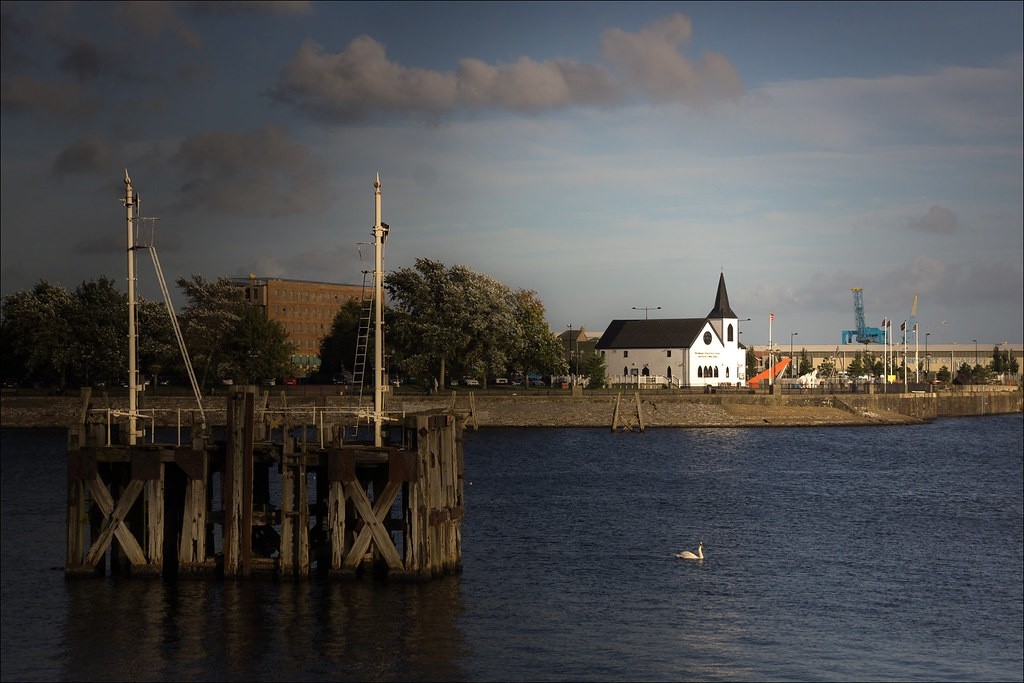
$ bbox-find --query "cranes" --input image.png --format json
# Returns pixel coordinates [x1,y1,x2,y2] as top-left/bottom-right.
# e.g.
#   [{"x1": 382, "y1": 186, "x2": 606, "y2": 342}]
[{"x1": 852, "y1": 288, "x2": 873, "y2": 343}]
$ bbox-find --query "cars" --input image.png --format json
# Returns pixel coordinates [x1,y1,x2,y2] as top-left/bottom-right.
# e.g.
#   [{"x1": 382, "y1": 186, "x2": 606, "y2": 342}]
[
  {"x1": 464, "y1": 377, "x2": 479, "y2": 387},
  {"x1": 496, "y1": 377, "x2": 547, "y2": 387},
  {"x1": 93, "y1": 378, "x2": 170, "y2": 389},
  {"x1": 281, "y1": 375, "x2": 297, "y2": 385},
  {"x1": 451, "y1": 378, "x2": 460, "y2": 386},
  {"x1": 221, "y1": 376, "x2": 234, "y2": 385},
  {"x1": 261, "y1": 375, "x2": 277, "y2": 388}
]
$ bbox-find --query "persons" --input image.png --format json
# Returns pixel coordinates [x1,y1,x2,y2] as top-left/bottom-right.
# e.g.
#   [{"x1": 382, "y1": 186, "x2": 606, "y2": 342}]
[
  {"x1": 433, "y1": 378, "x2": 438, "y2": 392},
  {"x1": 424, "y1": 378, "x2": 430, "y2": 392}
]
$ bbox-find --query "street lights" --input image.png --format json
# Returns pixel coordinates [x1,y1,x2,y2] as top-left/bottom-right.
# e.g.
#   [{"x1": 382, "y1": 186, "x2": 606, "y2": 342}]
[
  {"x1": 925, "y1": 353, "x2": 932, "y2": 373},
  {"x1": 632, "y1": 307, "x2": 661, "y2": 319},
  {"x1": 925, "y1": 332, "x2": 930, "y2": 371},
  {"x1": 972, "y1": 338, "x2": 978, "y2": 365},
  {"x1": 736, "y1": 318, "x2": 751, "y2": 348},
  {"x1": 789, "y1": 332, "x2": 798, "y2": 376},
  {"x1": 566, "y1": 322, "x2": 573, "y2": 388}
]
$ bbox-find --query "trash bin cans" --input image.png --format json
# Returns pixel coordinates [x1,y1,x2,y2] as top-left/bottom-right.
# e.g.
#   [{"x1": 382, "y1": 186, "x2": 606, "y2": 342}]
[
  {"x1": 737, "y1": 382, "x2": 741, "y2": 389},
  {"x1": 707, "y1": 385, "x2": 712, "y2": 394}
]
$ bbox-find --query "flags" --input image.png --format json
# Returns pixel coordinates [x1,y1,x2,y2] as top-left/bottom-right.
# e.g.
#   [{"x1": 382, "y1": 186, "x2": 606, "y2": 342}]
[
  {"x1": 900, "y1": 322, "x2": 905, "y2": 331},
  {"x1": 770, "y1": 312, "x2": 775, "y2": 322},
  {"x1": 912, "y1": 325, "x2": 916, "y2": 333},
  {"x1": 887, "y1": 320, "x2": 890, "y2": 326},
  {"x1": 882, "y1": 319, "x2": 885, "y2": 327}
]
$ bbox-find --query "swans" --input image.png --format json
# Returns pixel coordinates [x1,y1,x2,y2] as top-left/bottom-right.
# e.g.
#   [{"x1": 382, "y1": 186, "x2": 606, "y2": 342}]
[{"x1": 679, "y1": 541, "x2": 704, "y2": 559}]
